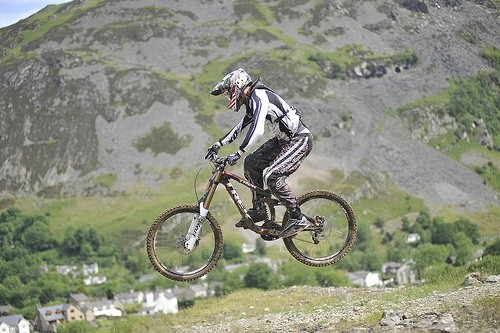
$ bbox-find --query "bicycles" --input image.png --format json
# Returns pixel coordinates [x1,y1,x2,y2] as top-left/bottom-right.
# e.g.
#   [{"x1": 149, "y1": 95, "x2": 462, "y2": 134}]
[{"x1": 146, "y1": 144, "x2": 357, "y2": 282}]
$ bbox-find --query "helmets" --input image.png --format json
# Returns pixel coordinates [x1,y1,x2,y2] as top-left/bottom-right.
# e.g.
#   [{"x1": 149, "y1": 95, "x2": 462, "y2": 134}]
[{"x1": 211, "y1": 67, "x2": 251, "y2": 111}]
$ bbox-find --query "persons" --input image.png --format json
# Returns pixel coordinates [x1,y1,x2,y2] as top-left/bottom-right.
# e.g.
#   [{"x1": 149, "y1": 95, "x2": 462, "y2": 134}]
[{"x1": 207, "y1": 67, "x2": 314, "y2": 238}]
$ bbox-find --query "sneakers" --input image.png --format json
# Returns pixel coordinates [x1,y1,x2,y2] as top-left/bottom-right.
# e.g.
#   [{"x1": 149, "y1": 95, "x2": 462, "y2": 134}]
[
  {"x1": 278, "y1": 216, "x2": 310, "y2": 239},
  {"x1": 235, "y1": 204, "x2": 268, "y2": 227}
]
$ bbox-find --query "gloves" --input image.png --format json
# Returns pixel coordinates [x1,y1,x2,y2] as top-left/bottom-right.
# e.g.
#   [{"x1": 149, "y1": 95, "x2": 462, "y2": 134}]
[
  {"x1": 206, "y1": 142, "x2": 220, "y2": 156},
  {"x1": 226, "y1": 151, "x2": 241, "y2": 166}
]
